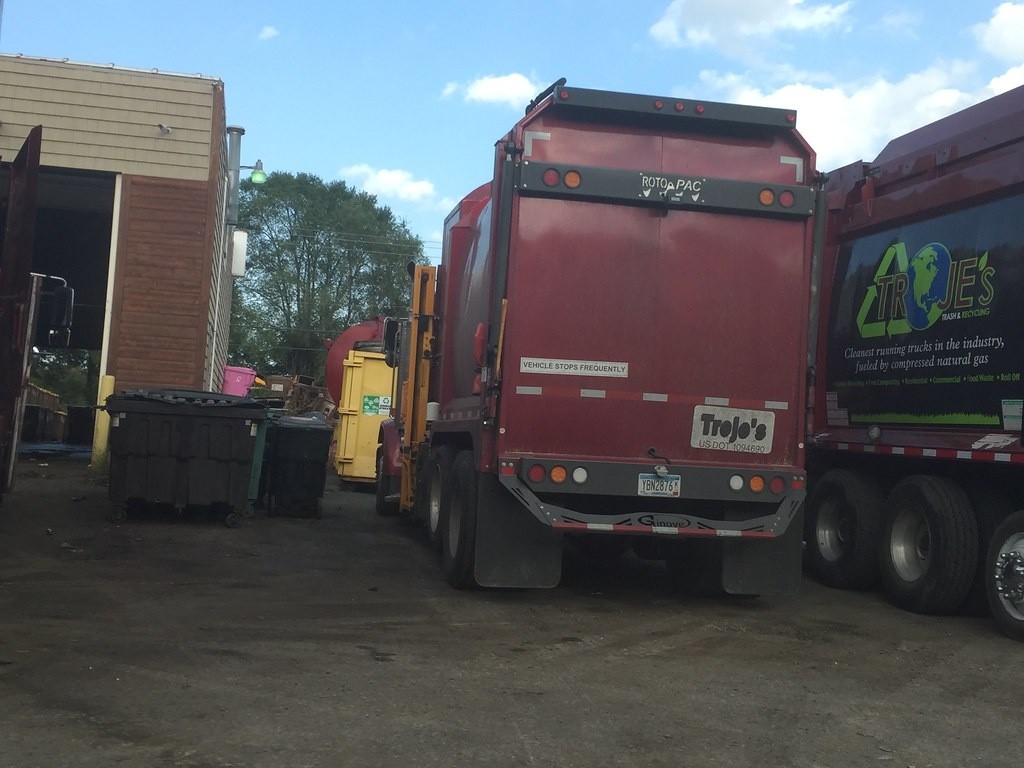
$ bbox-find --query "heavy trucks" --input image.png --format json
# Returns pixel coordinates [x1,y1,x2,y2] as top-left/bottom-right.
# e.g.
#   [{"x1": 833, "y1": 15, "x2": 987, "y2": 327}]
[
  {"x1": 801, "y1": 86, "x2": 1024, "y2": 635},
  {"x1": 370, "y1": 74, "x2": 821, "y2": 607}
]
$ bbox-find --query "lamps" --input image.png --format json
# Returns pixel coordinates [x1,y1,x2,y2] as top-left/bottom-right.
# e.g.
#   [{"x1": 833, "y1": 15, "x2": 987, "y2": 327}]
[{"x1": 250, "y1": 159, "x2": 267, "y2": 185}]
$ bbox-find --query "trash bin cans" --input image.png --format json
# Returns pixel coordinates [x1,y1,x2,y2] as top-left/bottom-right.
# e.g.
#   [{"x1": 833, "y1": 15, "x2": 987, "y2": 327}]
[{"x1": 107, "y1": 365, "x2": 335, "y2": 528}]
